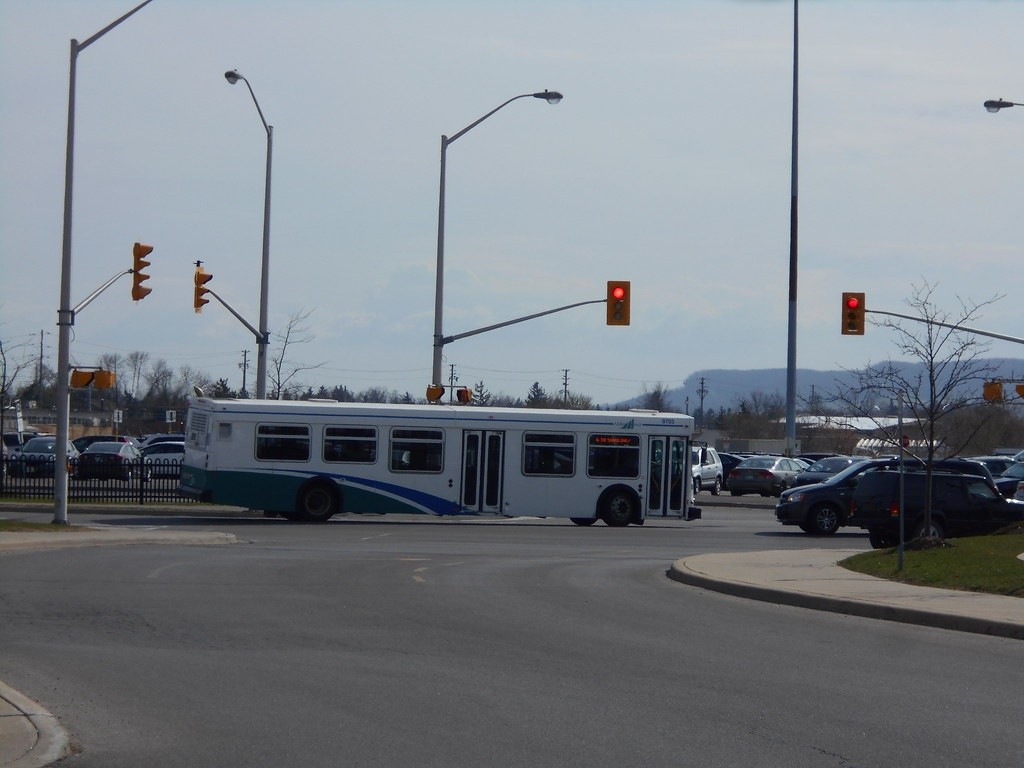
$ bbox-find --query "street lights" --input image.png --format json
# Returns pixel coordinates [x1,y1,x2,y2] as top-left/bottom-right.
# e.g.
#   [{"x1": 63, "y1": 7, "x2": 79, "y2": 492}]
[
  {"x1": 224, "y1": 71, "x2": 273, "y2": 399},
  {"x1": 431, "y1": 90, "x2": 563, "y2": 384}
]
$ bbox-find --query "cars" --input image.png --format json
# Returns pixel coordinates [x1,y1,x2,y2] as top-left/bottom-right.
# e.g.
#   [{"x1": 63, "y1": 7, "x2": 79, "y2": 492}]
[
  {"x1": 968, "y1": 448, "x2": 1024, "y2": 500},
  {"x1": 72, "y1": 436, "x2": 141, "y2": 453},
  {"x1": 716, "y1": 451, "x2": 848, "y2": 489},
  {"x1": 79, "y1": 442, "x2": 151, "y2": 482},
  {"x1": 661, "y1": 444, "x2": 723, "y2": 496},
  {"x1": 726, "y1": 455, "x2": 804, "y2": 498},
  {"x1": 791, "y1": 457, "x2": 873, "y2": 485},
  {"x1": 7, "y1": 437, "x2": 81, "y2": 475},
  {"x1": 534, "y1": 449, "x2": 593, "y2": 475},
  {"x1": 140, "y1": 441, "x2": 184, "y2": 475}
]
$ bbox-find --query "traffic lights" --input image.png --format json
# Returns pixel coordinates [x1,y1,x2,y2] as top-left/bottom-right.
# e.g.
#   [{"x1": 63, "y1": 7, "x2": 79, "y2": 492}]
[
  {"x1": 607, "y1": 282, "x2": 629, "y2": 325},
  {"x1": 427, "y1": 387, "x2": 445, "y2": 400},
  {"x1": 457, "y1": 389, "x2": 472, "y2": 402},
  {"x1": 131, "y1": 242, "x2": 153, "y2": 302},
  {"x1": 193, "y1": 262, "x2": 212, "y2": 313},
  {"x1": 71, "y1": 371, "x2": 94, "y2": 387},
  {"x1": 842, "y1": 292, "x2": 865, "y2": 334}
]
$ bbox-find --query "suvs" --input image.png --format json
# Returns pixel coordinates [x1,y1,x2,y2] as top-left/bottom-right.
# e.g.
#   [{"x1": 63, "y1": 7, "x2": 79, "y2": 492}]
[
  {"x1": 851, "y1": 470, "x2": 1024, "y2": 548},
  {"x1": 775, "y1": 458, "x2": 998, "y2": 537},
  {"x1": 2, "y1": 432, "x2": 41, "y2": 476},
  {"x1": 141, "y1": 434, "x2": 185, "y2": 448}
]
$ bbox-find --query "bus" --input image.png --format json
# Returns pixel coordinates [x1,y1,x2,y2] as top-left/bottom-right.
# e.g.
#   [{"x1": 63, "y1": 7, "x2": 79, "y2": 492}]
[{"x1": 177, "y1": 392, "x2": 706, "y2": 528}]
